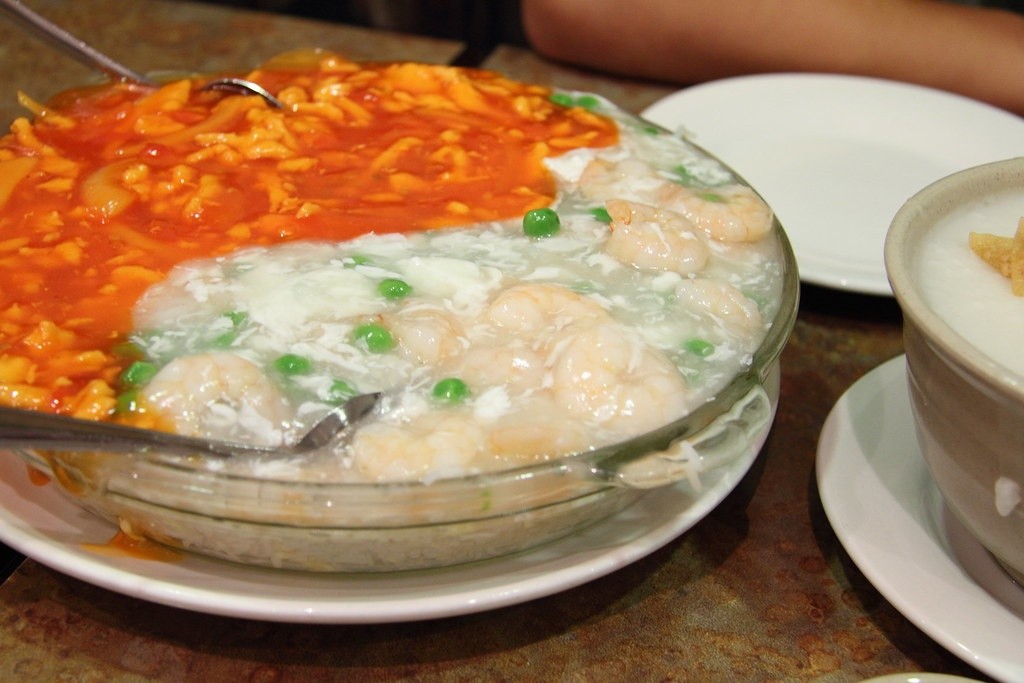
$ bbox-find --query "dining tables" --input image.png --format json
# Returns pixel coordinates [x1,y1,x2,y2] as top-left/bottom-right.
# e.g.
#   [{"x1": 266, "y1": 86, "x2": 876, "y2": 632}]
[{"x1": 0, "y1": 0, "x2": 1024, "y2": 682}]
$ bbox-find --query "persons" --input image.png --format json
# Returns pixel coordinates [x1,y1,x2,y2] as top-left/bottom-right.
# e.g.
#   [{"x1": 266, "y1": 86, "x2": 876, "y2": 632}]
[{"x1": 522, "y1": 0, "x2": 1023, "y2": 120}]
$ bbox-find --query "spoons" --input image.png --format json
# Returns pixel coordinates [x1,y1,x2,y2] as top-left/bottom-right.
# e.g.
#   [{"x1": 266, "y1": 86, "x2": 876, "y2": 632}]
[
  {"x1": 0, "y1": 0, "x2": 284, "y2": 119},
  {"x1": 1, "y1": 388, "x2": 380, "y2": 466}
]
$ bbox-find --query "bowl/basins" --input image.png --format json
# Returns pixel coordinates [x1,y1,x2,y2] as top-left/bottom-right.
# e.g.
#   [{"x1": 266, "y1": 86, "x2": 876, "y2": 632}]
[
  {"x1": 883, "y1": 156, "x2": 1024, "y2": 590},
  {"x1": 0, "y1": 65, "x2": 800, "y2": 583}
]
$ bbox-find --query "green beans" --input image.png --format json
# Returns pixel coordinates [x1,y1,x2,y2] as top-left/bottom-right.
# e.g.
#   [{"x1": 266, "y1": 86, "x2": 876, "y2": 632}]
[{"x1": 110, "y1": 91, "x2": 714, "y2": 415}]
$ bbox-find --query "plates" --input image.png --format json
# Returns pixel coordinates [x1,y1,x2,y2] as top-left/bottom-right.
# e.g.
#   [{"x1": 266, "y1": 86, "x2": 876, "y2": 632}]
[
  {"x1": 637, "y1": 72, "x2": 1024, "y2": 297},
  {"x1": 0, "y1": 348, "x2": 782, "y2": 626},
  {"x1": 813, "y1": 350, "x2": 1024, "y2": 683}
]
